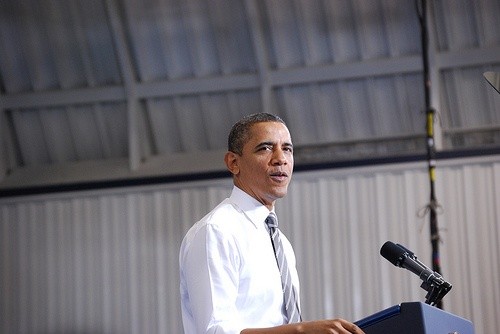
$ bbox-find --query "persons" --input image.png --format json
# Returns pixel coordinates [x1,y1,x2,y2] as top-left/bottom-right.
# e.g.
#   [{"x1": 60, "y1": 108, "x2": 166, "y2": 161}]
[{"x1": 179, "y1": 112, "x2": 364, "y2": 333}]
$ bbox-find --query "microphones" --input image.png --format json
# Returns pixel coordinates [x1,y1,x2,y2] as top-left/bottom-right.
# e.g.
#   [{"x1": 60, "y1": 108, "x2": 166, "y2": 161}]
[{"x1": 380, "y1": 241, "x2": 443, "y2": 286}]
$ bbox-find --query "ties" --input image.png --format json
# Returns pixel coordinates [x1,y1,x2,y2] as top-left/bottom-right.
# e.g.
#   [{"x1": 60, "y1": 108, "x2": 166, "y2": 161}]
[{"x1": 265, "y1": 216, "x2": 303, "y2": 324}]
